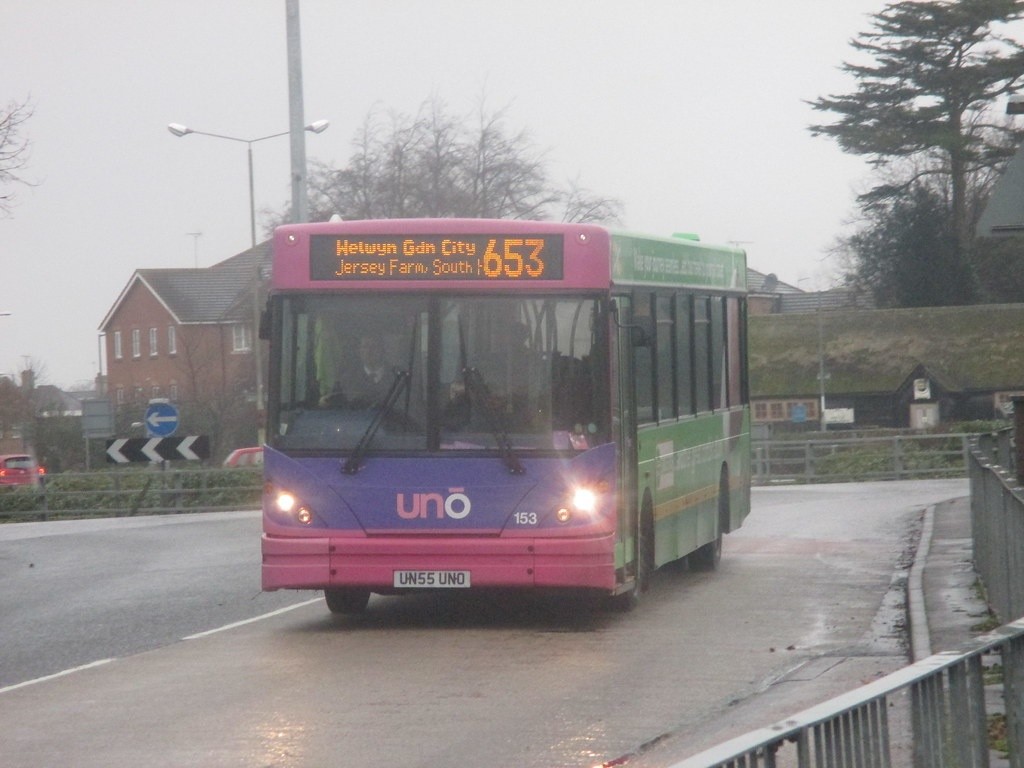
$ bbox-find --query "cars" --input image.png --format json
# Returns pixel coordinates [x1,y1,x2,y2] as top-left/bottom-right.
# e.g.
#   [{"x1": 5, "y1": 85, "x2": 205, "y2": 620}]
[{"x1": 1, "y1": 455, "x2": 46, "y2": 486}]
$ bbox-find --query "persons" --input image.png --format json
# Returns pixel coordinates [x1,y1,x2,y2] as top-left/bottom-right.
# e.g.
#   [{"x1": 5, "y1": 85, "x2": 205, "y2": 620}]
[{"x1": 318, "y1": 321, "x2": 532, "y2": 410}]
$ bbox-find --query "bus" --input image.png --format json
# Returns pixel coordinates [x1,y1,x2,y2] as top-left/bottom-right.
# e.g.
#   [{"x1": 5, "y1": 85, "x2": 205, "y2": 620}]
[{"x1": 257, "y1": 216, "x2": 760, "y2": 615}]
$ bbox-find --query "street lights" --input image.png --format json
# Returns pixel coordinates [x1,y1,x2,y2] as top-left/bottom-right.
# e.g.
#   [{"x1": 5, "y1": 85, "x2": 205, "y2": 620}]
[{"x1": 166, "y1": 121, "x2": 337, "y2": 462}]
